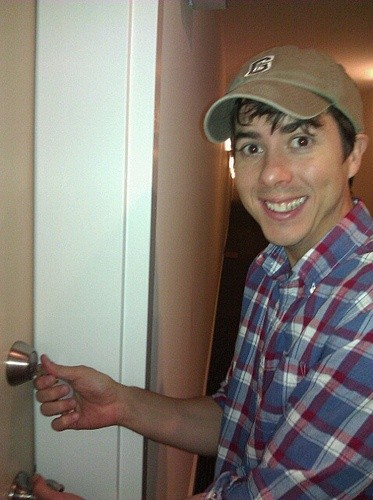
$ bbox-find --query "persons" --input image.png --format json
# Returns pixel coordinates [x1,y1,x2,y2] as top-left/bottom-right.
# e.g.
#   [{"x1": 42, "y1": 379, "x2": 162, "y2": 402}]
[{"x1": 29, "y1": 46, "x2": 373, "y2": 500}]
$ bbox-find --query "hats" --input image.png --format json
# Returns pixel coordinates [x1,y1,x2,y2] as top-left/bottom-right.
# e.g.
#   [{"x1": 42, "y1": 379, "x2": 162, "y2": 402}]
[{"x1": 202, "y1": 44, "x2": 364, "y2": 144}]
